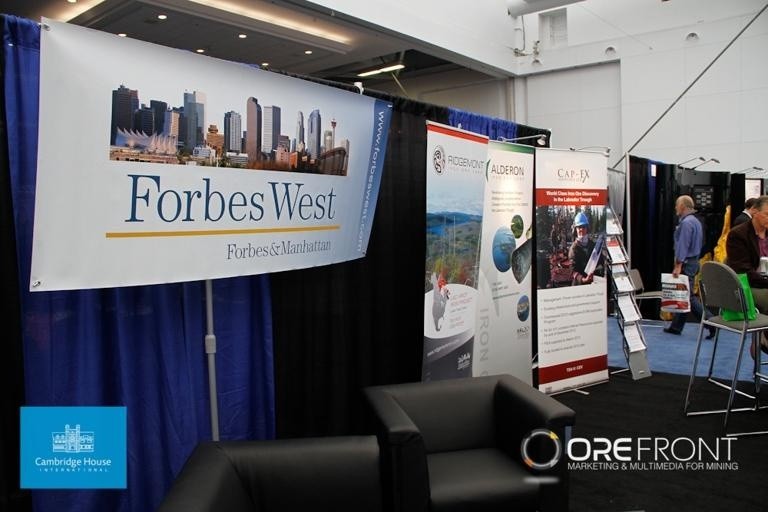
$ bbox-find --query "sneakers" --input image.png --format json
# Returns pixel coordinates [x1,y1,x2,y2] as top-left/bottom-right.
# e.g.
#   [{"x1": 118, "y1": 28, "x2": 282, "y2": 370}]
[
  {"x1": 705, "y1": 324, "x2": 716, "y2": 340},
  {"x1": 662, "y1": 326, "x2": 683, "y2": 335},
  {"x1": 750, "y1": 330, "x2": 767, "y2": 361}
]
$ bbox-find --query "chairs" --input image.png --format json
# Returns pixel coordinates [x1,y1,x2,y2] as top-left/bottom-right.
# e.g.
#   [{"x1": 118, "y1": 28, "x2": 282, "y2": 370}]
[
  {"x1": 366, "y1": 375, "x2": 576, "y2": 512},
  {"x1": 706, "y1": 306, "x2": 767, "y2": 405},
  {"x1": 683, "y1": 260, "x2": 767, "y2": 437},
  {"x1": 628, "y1": 269, "x2": 668, "y2": 328},
  {"x1": 156, "y1": 435, "x2": 380, "y2": 512}
]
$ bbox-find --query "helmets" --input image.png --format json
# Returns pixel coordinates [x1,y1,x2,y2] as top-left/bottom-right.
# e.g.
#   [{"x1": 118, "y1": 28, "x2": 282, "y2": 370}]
[{"x1": 573, "y1": 211, "x2": 590, "y2": 228}]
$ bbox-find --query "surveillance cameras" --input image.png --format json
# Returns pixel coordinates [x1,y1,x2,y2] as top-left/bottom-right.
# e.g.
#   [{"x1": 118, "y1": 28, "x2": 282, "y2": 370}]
[{"x1": 533, "y1": 39, "x2": 540, "y2": 44}]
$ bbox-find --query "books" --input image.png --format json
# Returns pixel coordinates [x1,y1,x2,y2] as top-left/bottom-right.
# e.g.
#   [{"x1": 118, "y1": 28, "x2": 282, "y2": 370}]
[{"x1": 607, "y1": 210, "x2": 648, "y2": 357}]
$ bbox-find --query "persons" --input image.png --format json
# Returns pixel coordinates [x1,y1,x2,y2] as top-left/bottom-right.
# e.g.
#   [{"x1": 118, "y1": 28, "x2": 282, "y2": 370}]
[
  {"x1": 550, "y1": 223, "x2": 562, "y2": 259},
  {"x1": 725, "y1": 194, "x2": 767, "y2": 358},
  {"x1": 567, "y1": 212, "x2": 604, "y2": 287},
  {"x1": 733, "y1": 198, "x2": 756, "y2": 228},
  {"x1": 664, "y1": 194, "x2": 716, "y2": 340}
]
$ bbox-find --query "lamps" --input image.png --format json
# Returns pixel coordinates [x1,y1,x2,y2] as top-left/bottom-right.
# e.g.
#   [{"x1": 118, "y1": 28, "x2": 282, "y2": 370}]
[
  {"x1": 356, "y1": 61, "x2": 405, "y2": 77},
  {"x1": 497, "y1": 134, "x2": 546, "y2": 145},
  {"x1": 678, "y1": 156, "x2": 764, "y2": 178},
  {"x1": 570, "y1": 145, "x2": 611, "y2": 154}
]
